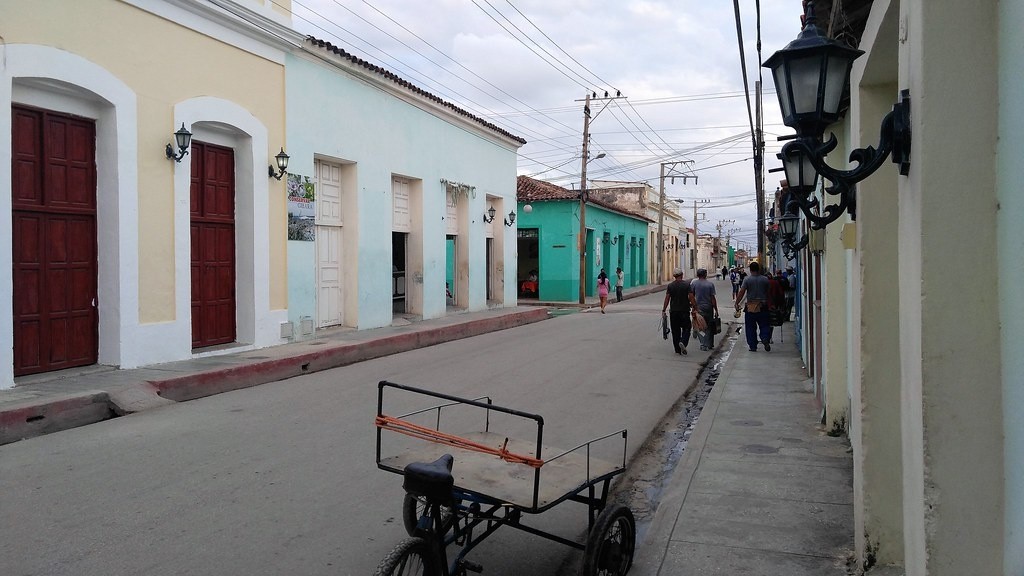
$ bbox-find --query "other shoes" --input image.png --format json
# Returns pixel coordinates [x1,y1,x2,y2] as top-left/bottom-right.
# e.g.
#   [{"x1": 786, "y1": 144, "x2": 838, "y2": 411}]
[
  {"x1": 700, "y1": 344, "x2": 708, "y2": 351},
  {"x1": 762, "y1": 339, "x2": 771, "y2": 352},
  {"x1": 749, "y1": 347, "x2": 756, "y2": 351},
  {"x1": 601, "y1": 309, "x2": 605, "y2": 314},
  {"x1": 733, "y1": 299, "x2": 736, "y2": 302},
  {"x1": 679, "y1": 341, "x2": 689, "y2": 357}
]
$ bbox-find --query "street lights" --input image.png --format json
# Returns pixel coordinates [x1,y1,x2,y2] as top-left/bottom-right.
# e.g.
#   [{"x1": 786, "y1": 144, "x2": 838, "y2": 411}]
[
  {"x1": 656, "y1": 199, "x2": 684, "y2": 285},
  {"x1": 579, "y1": 153, "x2": 607, "y2": 305},
  {"x1": 694, "y1": 220, "x2": 710, "y2": 278}
]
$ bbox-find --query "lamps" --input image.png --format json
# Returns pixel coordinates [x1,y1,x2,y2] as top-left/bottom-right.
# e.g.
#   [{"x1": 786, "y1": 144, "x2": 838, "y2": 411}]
[
  {"x1": 484, "y1": 205, "x2": 496, "y2": 223},
  {"x1": 268, "y1": 147, "x2": 290, "y2": 181},
  {"x1": 779, "y1": 209, "x2": 809, "y2": 261},
  {"x1": 504, "y1": 210, "x2": 516, "y2": 227},
  {"x1": 630, "y1": 240, "x2": 643, "y2": 248},
  {"x1": 776, "y1": 136, "x2": 856, "y2": 232},
  {"x1": 166, "y1": 122, "x2": 192, "y2": 163},
  {"x1": 602, "y1": 234, "x2": 609, "y2": 244},
  {"x1": 611, "y1": 236, "x2": 619, "y2": 246},
  {"x1": 761, "y1": 0, "x2": 912, "y2": 195}
]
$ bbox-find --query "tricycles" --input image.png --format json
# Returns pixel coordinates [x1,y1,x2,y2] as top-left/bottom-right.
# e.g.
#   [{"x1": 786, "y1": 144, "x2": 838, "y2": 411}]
[{"x1": 371, "y1": 381, "x2": 637, "y2": 576}]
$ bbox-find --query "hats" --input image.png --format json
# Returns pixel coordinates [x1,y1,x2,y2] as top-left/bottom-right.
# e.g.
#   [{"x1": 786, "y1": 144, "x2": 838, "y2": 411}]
[
  {"x1": 673, "y1": 268, "x2": 683, "y2": 277},
  {"x1": 784, "y1": 266, "x2": 793, "y2": 271}
]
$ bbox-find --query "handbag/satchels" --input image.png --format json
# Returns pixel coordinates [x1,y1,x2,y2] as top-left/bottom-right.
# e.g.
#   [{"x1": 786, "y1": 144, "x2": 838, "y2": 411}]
[
  {"x1": 743, "y1": 301, "x2": 760, "y2": 313},
  {"x1": 771, "y1": 307, "x2": 784, "y2": 328},
  {"x1": 711, "y1": 316, "x2": 722, "y2": 335}
]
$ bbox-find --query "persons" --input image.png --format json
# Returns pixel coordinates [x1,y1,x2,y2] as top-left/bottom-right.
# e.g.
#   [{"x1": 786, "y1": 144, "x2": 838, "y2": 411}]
[
  {"x1": 662, "y1": 269, "x2": 699, "y2": 356},
  {"x1": 728, "y1": 262, "x2": 796, "y2": 352},
  {"x1": 596, "y1": 268, "x2": 611, "y2": 315},
  {"x1": 615, "y1": 267, "x2": 625, "y2": 302},
  {"x1": 716, "y1": 266, "x2": 728, "y2": 280},
  {"x1": 529, "y1": 272, "x2": 537, "y2": 282},
  {"x1": 446, "y1": 282, "x2": 453, "y2": 298},
  {"x1": 689, "y1": 269, "x2": 719, "y2": 351}
]
